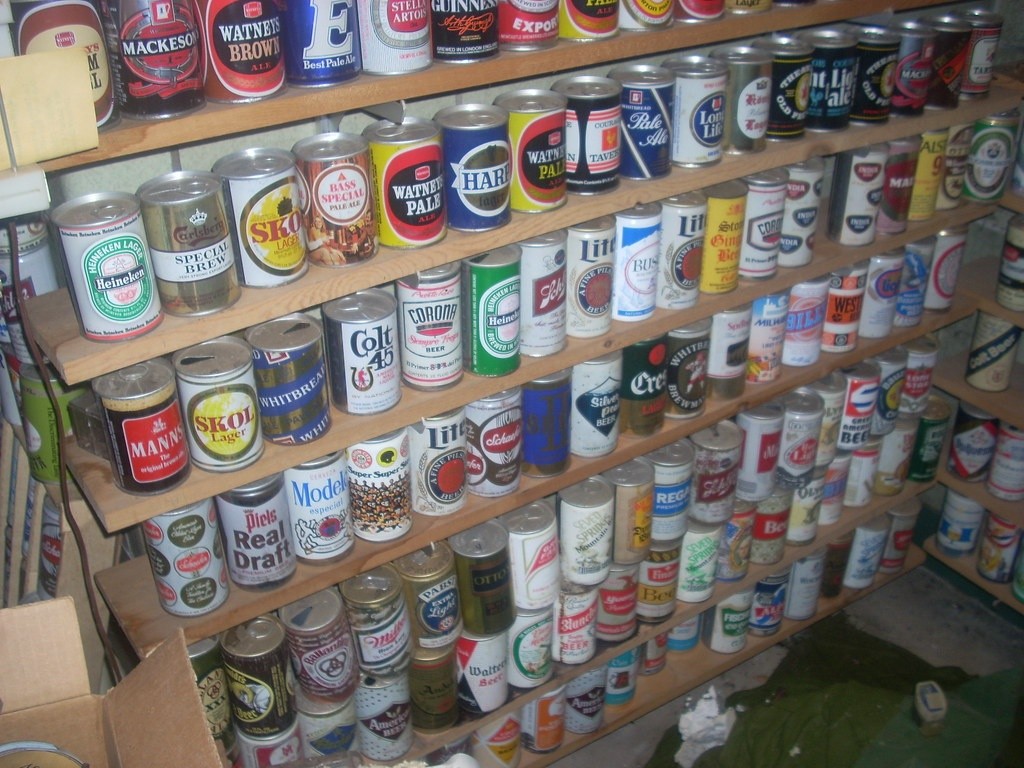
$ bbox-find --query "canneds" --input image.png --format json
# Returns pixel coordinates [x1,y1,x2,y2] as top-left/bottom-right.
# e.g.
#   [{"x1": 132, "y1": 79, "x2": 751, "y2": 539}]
[{"x1": 0, "y1": 0, "x2": 1024, "y2": 768}]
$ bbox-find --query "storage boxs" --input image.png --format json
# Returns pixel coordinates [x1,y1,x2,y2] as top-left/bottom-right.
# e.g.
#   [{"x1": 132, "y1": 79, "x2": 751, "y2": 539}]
[{"x1": 0, "y1": 591, "x2": 229, "y2": 768}]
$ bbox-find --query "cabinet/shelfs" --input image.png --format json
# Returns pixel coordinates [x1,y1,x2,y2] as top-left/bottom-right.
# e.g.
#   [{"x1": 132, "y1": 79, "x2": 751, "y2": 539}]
[{"x1": 0, "y1": 0, "x2": 1024, "y2": 768}]
